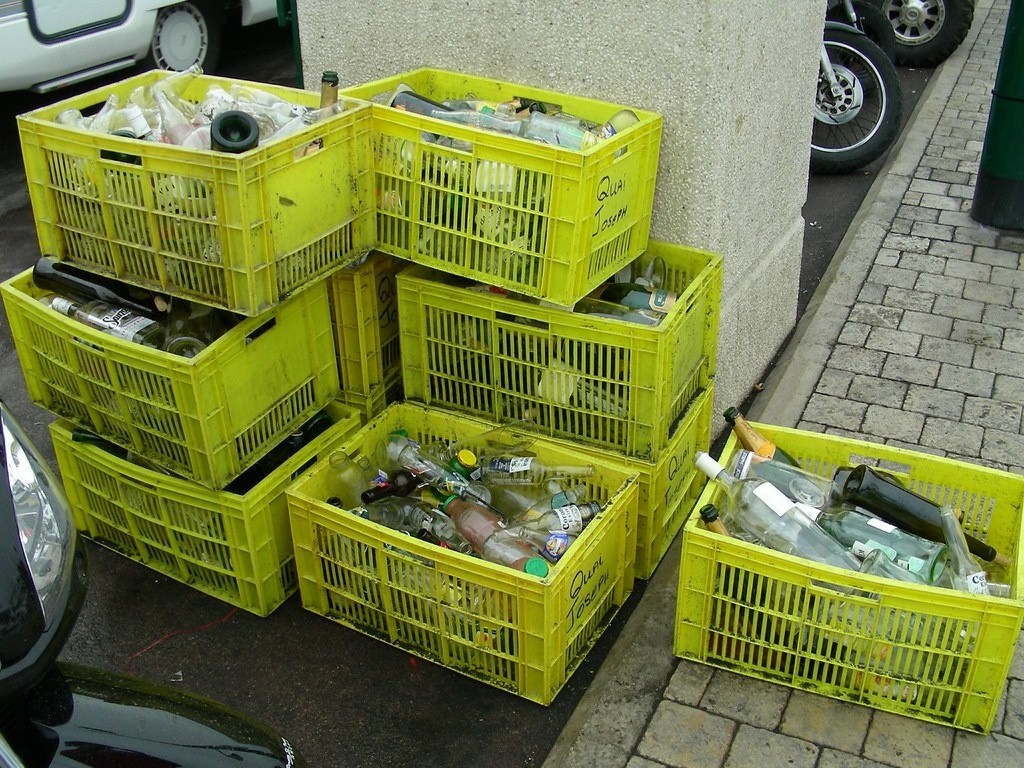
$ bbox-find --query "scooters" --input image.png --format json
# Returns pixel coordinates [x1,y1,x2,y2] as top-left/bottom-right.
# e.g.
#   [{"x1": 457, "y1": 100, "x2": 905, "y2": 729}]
[{"x1": 0, "y1": 395, "x2": 314, "y2": 768}]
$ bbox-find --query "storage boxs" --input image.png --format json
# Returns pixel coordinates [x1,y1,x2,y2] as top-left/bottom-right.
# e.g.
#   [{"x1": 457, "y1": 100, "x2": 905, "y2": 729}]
[
  {"x1": 327, "y1": 252, "x2": 409, "y2": 393},
  {"x1": 537, "y1": 384, "x2": 718, "y2": 578},
  {"x1": 13, "y1": 70, "x2": 379, "y2": 314},
  {"x1": 0, "y1": 266, "x2": 344, "y2": 486},
  {"x1": 46, "y1": 400, "x2": 361, "y2": 618},
  {"x1": 671, "y1": 421, "x2": 1024, "y2": 736},
  {"x1": 394, "y1": 239, "x2": 725, "y2": 459},
  {"x1": 334, "y1": 366, "x2": 403, "y2": 428},
  {"x1": 339, "y1": 66, "x2": 665, "y2": 305},
  {"x1": 285, "y1": 401, "x2": 641, "y2": 709}
]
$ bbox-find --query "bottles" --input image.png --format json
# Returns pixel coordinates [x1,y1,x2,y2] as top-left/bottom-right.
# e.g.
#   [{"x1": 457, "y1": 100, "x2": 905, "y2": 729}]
[
  {"x1": 692, "y1": 407, "x2": 1020, "y2": 724},
  {"x1": 18, "y1": 66, "x2": 721, "y2": 415},
  {"x1": 46, "y1": 422, "x2": 602, "y2": 694}
]
